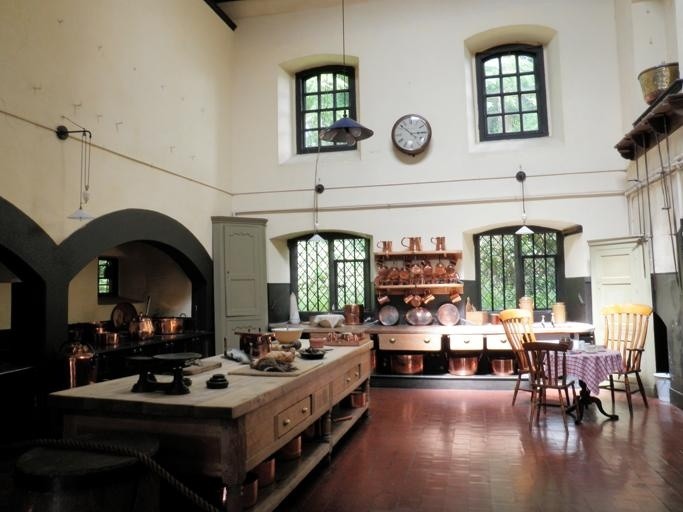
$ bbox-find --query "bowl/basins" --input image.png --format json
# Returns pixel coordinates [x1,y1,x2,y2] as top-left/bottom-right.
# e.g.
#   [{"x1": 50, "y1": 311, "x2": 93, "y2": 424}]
[
  {"x1": 567, "y1": 338, "x2": 599, "y2": 356},
  {"x1": 288, "y1": 318, "x2": 301, "y2": 326},
  {"x1": 388, "y1": 353, "x2": 426, "y2": 376},
  {"x1": 218, "y1": 476, "x2": 257, "y2": 509},
  {"x1": 309, "y1": 336, "x2": 326, "y2": 348},
  {"x1": 348, "y1": 388, "x2": 367, "y2": 408},
  {"x1": 490, "y1": 357, "x2": 514, "y2": 378},
  {"x1": 249, "y1": 458, "x2": 276, "y2": 487},
  {"x1": 445, "y1": 357, "x2": 480, "y2": 376},
  {"x1": 314, "y1": 314, "x2": 344, "y2": 328},
  {"x1": 405, "y1": 306, "x2": 432, "y2": 326},
  {"x1": 273, "y1": 327, "x2": 303, "y2": 347},
  {"x1": 275, "y1": 434, "x2": 302, "y2": 459},
  {"x1": 435, "y1": 302, "x2": 460, "y2": 326},
  {"x1": 300, "y1": 349, "x2": 325, "y2": 360}
]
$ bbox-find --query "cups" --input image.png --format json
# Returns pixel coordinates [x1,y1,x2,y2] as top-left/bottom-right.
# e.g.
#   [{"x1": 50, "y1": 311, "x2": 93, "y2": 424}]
[
  {"x1": 400, "y1": 235, "x2": 423, "y2": 253},
  {"x1": 429, "y1": 236, "x2": 448, "y2": 252},
  {"x1": 402, "y1": 287, "x2": 435, "y2": 306},
  {"x1": 96, "y1": 327, "x2": 103, "y2": 335},
  {"x1": 376, "y1": 239, "x2": 393, "y2": 254},
  {"x1": 447, "y1": 289, "x2": 460, "y2": 304},
  {"x1": 490, "y1": 313, "x2": 500, "y2": 325},
  {"x1": 376, "y1": 288, "x2": 392, "y2": 305},
  {"x1": 335, "y1": 332, "x2": 360, "y2": 344},
  {"x1": 105, "y1": 332, "x2": 119, "y2": 345}
]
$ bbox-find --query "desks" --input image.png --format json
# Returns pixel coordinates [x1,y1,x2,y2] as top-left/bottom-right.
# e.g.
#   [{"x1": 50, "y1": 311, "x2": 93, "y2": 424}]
[
  {"x1": 49, "y1": 339, "x2": 375, "y2": 512},
  {"x1": 55, "y1": 329, "x2": 214, "y2": 388},
  {"x1": 530, "y1": 321, "x2": 596, "y2": 339}
]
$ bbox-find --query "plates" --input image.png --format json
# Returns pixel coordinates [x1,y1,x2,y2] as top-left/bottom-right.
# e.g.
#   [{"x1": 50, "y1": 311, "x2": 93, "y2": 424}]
[
  {"x1": 378, "y1": 304, "x2": 399, "y2": 326},
  {"x1": 303, "y1": 321, "x2": 311, "y2": 325}
]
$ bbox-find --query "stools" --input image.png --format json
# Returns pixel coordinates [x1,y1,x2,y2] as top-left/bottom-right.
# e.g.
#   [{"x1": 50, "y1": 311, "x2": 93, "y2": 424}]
[{"x1": 13, "y1": 434, "x2": 160, "y2": 512}]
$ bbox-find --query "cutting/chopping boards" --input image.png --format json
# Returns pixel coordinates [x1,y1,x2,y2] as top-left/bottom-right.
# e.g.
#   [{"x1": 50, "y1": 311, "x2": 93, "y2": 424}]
[
  {"x1": 226, "y1": 359, "x2": 324, "y2": 377},
  {"x1": 183, "y1": 358, "x2": 222, "y2": 375}
]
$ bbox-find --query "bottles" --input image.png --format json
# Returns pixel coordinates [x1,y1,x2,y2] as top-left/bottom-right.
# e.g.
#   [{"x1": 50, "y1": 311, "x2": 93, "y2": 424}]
[
  {"x1": 552, "y1": 302, "x2": 566, "y2": 325},
  {"x1": 519, "y1": 296, "x2": 534, "y2": 326}
]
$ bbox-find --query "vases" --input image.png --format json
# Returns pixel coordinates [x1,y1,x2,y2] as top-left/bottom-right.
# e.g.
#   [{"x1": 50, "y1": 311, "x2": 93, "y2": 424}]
[{"x1": 638, "y1": 62, "x2": 679, "y2": 105}]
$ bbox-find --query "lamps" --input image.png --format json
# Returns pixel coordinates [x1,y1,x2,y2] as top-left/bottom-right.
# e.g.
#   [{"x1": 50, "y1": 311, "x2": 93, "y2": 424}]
[
  {"x1": 53, "y1": 125, "x2": 97, "y2": 220},
  {"x1": 307, "y1": 184, "x2": 327, "y2": 243},
  {"x1": 513, "y1": 171, "x2": 535, "y2": 234},
  {"x1": 318, "y1": 1, "x2": 374, "y2": 146}
]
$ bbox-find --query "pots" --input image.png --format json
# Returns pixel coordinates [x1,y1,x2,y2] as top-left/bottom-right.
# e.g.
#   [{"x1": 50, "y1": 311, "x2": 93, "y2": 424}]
[
  {"x1": 374, "y1": 255, "x2": 461, "y2": 285},
  {"x1": 240, "y1": 333, "x2": 271, "y2": 358},
  {"x1": 157, "y1": 313, "x2": 185, "y2": 334}
]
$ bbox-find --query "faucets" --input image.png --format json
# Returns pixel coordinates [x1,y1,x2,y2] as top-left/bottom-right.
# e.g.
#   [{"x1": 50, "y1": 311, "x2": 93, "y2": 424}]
[
  {"x1": 539, "y1": 315, "x2": 546, "y2": 328},
  {"x1": 550, "y1": 313, "x2": 556, "y2": 328}
]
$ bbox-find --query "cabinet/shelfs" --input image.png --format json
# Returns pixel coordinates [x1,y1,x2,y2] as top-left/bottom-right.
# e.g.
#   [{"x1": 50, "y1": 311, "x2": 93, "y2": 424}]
[{"x1": 375, "y1": 251, "x2": 464, "y2": 295}]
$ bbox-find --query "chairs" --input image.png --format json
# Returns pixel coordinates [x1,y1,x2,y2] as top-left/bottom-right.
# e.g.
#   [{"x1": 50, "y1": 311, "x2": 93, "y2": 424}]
[
  {"x1": 598, "y1": 303, "x2": 654, "y2": 421},
  {"x1": 522, "y1": 342, "x2": 580, "y2": 435},
  {"x1": 497, "y1": 309, "x2": 549, "y2": 406}
]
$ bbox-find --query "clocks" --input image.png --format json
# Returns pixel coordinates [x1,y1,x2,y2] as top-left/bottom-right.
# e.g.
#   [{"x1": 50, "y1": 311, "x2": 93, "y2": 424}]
[{"x1": 391, "y1": 114, "x2": 432, "y2": 157}]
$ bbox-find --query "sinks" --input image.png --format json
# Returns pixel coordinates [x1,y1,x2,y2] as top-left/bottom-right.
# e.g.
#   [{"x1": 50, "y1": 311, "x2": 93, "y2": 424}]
[{"x1": 532, "y1": 324, "x2": 576, "y2": 328}]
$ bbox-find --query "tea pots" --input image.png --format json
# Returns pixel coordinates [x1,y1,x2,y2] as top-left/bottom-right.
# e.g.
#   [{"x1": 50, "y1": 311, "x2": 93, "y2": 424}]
[{"x1": 129, "y1": 311, "x2": 155, "y2": 340}]
